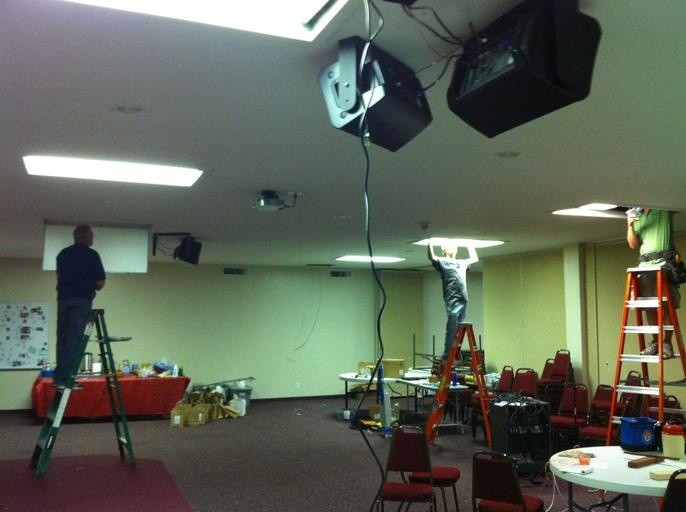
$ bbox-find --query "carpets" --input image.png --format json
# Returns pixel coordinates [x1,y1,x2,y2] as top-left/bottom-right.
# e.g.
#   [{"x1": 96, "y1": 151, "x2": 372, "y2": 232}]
[{"x1": 0, "y1": 455, "x2": 195, "y2": 512}]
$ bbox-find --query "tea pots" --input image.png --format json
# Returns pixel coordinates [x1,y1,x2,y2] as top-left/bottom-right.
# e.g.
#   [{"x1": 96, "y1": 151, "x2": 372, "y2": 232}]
[
  {"x1": 97, "y1": 351, "x2": 113, "y2": 371},
  {"x1": 80, "y1": 352, "x2": 93, "y2": 374}
]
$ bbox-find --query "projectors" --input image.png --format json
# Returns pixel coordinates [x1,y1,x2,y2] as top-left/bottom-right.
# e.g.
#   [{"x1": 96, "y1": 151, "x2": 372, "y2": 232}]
[{"x1": 254, "y1": 198, "x2": 285, "y2": 211}]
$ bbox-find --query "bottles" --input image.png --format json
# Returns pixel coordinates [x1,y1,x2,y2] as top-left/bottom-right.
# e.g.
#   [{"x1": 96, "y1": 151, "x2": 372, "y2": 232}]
[
  {"x1": 121, "y1": 358, "x2": 130, "y2": 368},
  {"x1": 178, "y1": 360, "x2": 185, "y2": 375},
  {"x1": 171, "y1": 364, "x2": 178, "y2": 376},
  {"x1": 41, "y1": 361, "x2": 54, "y2": 376}
]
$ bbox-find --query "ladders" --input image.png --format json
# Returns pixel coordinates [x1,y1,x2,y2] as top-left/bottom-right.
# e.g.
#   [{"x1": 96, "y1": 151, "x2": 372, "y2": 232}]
[
  {"x1": 30, "y1": 309, "x2": 137, "y2": 477},
  {"x1": 598, "y1": 267, "x2": 686, "y2": 511},
  {"x1": 425, "y1": 322, "x2": 493, "y2": 450}
]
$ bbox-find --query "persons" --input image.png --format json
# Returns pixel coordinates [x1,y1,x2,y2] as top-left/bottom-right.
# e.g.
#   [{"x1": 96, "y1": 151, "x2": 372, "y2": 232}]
[
  {"x1": 428, "y1": 242, "x2": 479, "y2": 363},
  {"x1": 53, "y1": 225, "x2": 106, "y2": 390},
  {"x1": 627, "y1": 208, "x2": 680, "y2": 359}
]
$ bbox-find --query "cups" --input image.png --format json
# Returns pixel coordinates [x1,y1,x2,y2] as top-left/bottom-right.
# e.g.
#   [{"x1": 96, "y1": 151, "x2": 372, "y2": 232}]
[{"x1": 660, "y1": 423, "x2": 685, "y2": 459}]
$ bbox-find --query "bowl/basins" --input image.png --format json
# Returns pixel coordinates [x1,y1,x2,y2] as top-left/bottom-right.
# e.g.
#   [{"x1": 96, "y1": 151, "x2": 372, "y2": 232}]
[{"x1": 151, "y1": 360, "x2": 169, "y2": 372}]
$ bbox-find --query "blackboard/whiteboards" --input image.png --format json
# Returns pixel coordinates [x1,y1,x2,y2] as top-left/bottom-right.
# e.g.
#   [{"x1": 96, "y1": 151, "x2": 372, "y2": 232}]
[{"x1": 0, "y1": 302, "x2": 50, "y2": 371}]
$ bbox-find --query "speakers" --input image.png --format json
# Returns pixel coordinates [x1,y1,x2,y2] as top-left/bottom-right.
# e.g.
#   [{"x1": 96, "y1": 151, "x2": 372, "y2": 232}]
[
  {"x1": 446, "y1": 7, "x2": 603, "y2": 139},
  {"x1": 175, "y1": 240, "x2": 202, "y2": 264},
  {"x1": 319, "y1": 51, "x2": 434, "y2": 152}
]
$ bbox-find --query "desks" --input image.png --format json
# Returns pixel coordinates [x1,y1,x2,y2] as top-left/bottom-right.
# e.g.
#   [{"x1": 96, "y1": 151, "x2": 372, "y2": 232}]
[
  {"x1": 31, "y1": 376, "x2": 190, "y2": 418},
  {"x1": 339, "y1": 372, "x2": 399, "y2": 409}
]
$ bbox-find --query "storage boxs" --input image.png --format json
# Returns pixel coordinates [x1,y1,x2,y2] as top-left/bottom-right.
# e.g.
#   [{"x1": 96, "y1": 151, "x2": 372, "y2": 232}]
[{"x1": 229, "y1": 384, "x2": 252, "y2": 408}]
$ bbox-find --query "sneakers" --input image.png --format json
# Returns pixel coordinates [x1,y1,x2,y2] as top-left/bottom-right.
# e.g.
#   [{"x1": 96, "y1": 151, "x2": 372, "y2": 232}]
[
  {"x1": 663, "y1": 343, "x2": 673, "y2": 359},
  {"x1": 52, "y1": 381, "x2": 83, "y2": 389},
  {"x1": 640, "y1": 341, "x2": 658, "y2": 354}
]
